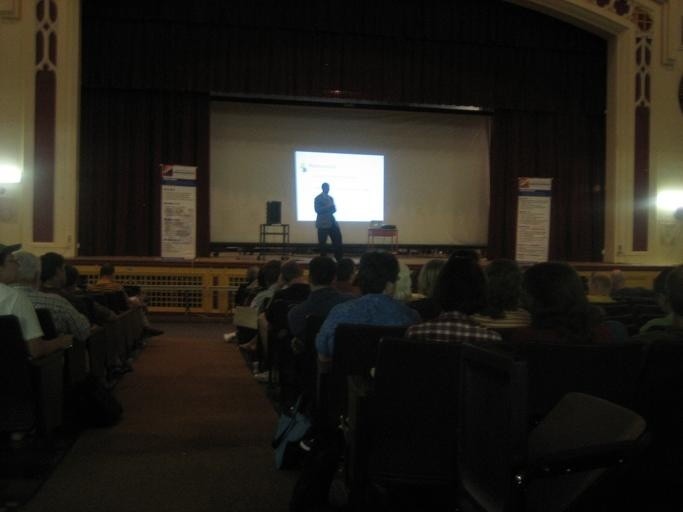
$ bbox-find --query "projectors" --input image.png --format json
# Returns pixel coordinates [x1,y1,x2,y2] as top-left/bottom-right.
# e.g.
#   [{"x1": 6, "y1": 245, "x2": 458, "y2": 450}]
[{"x1": 382, "y1": 225, "x2": 396, "y2": 229}]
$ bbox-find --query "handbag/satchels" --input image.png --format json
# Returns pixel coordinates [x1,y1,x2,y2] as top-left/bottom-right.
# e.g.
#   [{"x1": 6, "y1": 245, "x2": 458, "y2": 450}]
[{"x1": 70, "y1": 375, "x2": 123, "y2": 429}]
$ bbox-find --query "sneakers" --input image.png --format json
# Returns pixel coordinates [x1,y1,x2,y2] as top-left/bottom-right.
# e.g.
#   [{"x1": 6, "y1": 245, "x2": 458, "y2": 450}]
[
  {"x1": 253, "y1": 372, "x2": 270, "y2": 381},
  {"x1": 142, "y1": 326, "x2": 166, "y2": 339}
]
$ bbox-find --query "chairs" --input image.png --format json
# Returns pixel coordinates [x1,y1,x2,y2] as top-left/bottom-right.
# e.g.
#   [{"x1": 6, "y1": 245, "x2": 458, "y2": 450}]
[{"x1": 0, "y1": 280, "x2": 152, "y2": 488}]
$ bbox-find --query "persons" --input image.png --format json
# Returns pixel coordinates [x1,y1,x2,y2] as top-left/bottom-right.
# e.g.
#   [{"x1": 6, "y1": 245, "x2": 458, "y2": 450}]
[
  {"x1": 0, "y1": 242, "x2": 164, "y2": 429},
  {"x1": 312, "y1": 181, "x2": 344, "y2": 261},
  {"x1": 233, "y1": 241, "x2": 682, "y2": 510}
]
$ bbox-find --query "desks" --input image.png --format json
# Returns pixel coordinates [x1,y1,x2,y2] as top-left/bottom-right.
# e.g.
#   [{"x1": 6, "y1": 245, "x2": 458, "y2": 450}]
[
  {"x1": 255, "y1": 221, "x2": 294, "y2": 261},
  {"x1": 365, "y1": 226, "x2": 399, "y2": 252}
]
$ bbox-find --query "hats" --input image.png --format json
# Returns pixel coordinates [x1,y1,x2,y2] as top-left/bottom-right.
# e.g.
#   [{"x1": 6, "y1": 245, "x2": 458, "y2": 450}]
[{"x1": 0, "y1": 243, "x2": 22, "y2": 254}]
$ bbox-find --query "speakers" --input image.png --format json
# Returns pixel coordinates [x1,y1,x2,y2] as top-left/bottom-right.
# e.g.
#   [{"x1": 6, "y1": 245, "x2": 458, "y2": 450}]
[{"x1": 267, "y1": 201, "x2": 281, "y2": 224}]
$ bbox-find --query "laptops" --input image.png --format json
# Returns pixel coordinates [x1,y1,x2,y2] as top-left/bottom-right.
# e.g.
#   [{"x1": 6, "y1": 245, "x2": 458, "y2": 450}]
[{"x1": 368, "y1": 221, "x2": 383, "y2": 228}]
